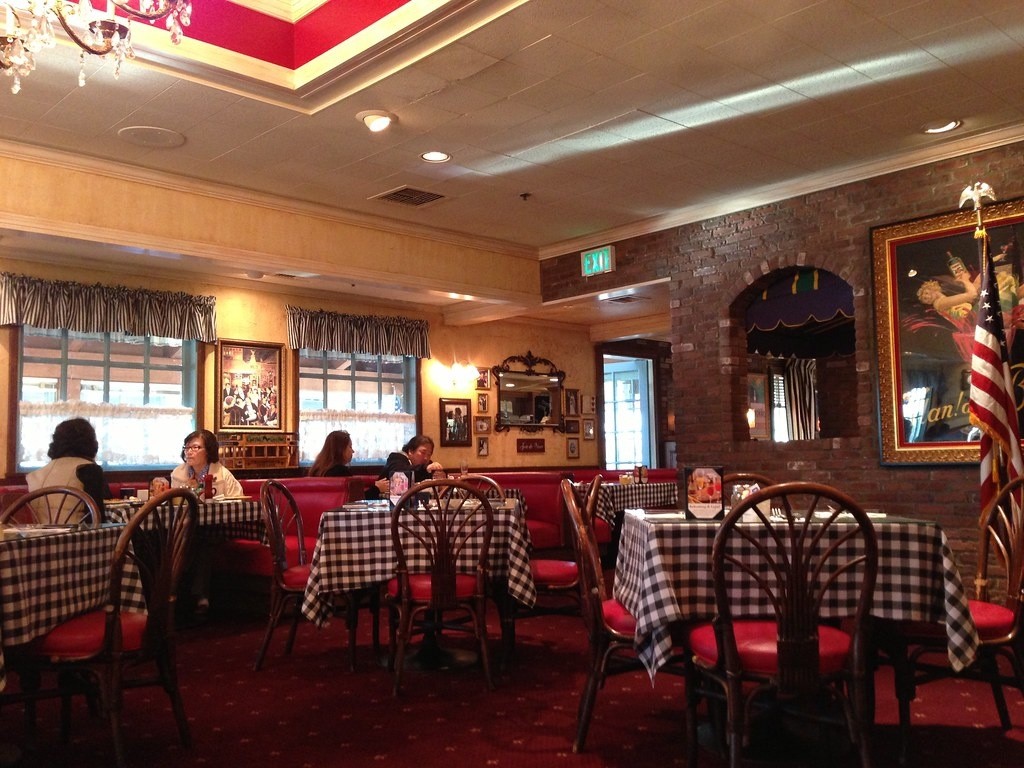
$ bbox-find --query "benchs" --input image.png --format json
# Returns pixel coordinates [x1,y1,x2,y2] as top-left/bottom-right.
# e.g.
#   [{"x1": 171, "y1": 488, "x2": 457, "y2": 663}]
[{"x1": 0, "y1": 466, "x2": 678, "y2": 662}]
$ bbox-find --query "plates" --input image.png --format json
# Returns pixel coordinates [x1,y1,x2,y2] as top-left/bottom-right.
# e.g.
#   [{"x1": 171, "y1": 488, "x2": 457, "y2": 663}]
[
  {"x1": 103, "y1": 500, "x2": 133, "y2": 503},
  {"x1": 814, "y1": 510, "x2": 886, "y2": 522}
]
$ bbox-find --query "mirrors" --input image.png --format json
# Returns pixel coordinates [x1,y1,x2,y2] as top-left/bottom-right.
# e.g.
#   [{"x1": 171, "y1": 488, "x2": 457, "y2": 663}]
[{"x1": 491, "y1": 351, "x2": 567, "y2": 433}]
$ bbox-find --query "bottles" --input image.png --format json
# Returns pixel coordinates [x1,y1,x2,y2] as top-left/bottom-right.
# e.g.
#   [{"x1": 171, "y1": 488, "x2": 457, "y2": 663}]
[
  {"x1": 619, "y1": 472, "x2": 634, "y2": 484},
  {"x1": 190, "y1": 478, "x2": 206, "y2": 502}
]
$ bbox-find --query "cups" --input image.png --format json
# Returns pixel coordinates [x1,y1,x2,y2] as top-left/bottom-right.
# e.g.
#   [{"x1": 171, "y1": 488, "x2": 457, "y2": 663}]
[
  {"x1": 506, "y1": 498, "x2": 516, "y2": 508},
  {"x1": 461, "y1": 463, "x2": 468, "y2": 475}
]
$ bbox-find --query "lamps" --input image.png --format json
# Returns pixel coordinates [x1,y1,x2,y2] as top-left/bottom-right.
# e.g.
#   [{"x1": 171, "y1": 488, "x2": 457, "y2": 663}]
[
  {"x1": 437, "y1": 363, "x2": 482, "y2": 393},
  {"x1": 0, "y1": 0, "x2": 192, "y2": 94},
  {"x1": 365, "y1": 115, "x2": 390, "y2": 132}
]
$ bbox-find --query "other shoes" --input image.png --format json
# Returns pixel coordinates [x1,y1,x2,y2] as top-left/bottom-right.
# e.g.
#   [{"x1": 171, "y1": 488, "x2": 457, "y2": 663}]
[{"x1": 194, "y1": 601, "x2": 210, "y2": 613}]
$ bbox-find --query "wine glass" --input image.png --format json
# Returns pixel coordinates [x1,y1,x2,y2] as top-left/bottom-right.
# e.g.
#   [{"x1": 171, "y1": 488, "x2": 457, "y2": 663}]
[{"x1": 688, "y1": 469, "x2": 721, "y2": 504}]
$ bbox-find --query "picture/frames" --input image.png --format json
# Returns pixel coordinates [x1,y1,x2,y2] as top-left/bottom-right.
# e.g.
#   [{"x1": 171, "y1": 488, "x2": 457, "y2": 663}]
[
  {"x1": 438, "y1": 368, "x2": 600, "y2": 457},
  {"x1": 868, "y1": 196, "x2": 1024, "y2": 467},
  {"x1": 215, "y1": 339, "x2": 285, "y2": 433},
  {"x1": 745, "y1": 373, "x2": 771, "y2": 439}
]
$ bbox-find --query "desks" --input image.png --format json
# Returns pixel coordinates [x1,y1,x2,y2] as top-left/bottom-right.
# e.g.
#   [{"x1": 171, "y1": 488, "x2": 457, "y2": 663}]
[
  {"x1": 614, "y1": 506, "x2": 980, "y2": 762},
  {"x1": 573, "y1": 481, "x2": 678, "y2": 529},
  {"x1": 380, "y1": 489, "x2": 534, "y2": 554},
  {"x1": 103, "y1": 500, "x2": 271, "y2": 638},
  {"x1": 0, "y1": 520, "x2": 148, "y2": 768},
  {"x1": 301, "y1": 498, "x2": 537, "y2": 674}
]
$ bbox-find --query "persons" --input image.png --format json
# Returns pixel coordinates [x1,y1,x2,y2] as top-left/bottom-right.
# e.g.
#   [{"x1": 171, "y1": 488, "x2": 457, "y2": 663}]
[
  {"x1": 223, "y1": 380, "x2": 277, "y2": 425},
  {"x1": 170, "y1": 429, "x2": 244, "y2": 497},
  {"x1": 378, "y1": 435, "x2": 443, "y2": 488},
  {"x1": 25, "y1": 418, "x2": 108, "y2": 524},
  {"x1": 308, "y1": 431, "x2": 389, "y2": 500}
]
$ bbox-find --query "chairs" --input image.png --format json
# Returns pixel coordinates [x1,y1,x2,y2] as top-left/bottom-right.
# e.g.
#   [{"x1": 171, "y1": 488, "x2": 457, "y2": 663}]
[
  {"x1": 252, "y1": 478, "x2": 380, "y2": 669},
  {"x1": 384, "y1": 475, "x2": 1024, "y2": 768},
  {"x1": 6, "y1": 487, "x2": 198, "y2": 767}
]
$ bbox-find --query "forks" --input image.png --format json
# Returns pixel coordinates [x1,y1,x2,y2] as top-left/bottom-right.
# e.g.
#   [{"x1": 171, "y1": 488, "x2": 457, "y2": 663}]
[{"x1": 772, "y1": 508, "x2": 781, "y2": 518}]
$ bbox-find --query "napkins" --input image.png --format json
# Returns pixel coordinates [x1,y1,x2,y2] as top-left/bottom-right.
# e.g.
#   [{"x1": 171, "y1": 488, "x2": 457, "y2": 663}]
[
  {"x1": 769, "y1": 514, "x2": 811, "y2": 524},
  {"x1": 815, "y1": 511, "x2": 886, "y2": 519},
  {"x1": 3, "y1": 528, "x2": 72, "y2": 538},
  {"x1": 624, "y1": 508, "x2": 687, "y2": 519}
]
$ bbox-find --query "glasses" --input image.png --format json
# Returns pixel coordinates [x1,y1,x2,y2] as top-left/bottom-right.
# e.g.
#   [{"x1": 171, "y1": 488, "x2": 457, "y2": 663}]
[{"x1": 183, "y1": 445, "x2": 206, "y2": 452}]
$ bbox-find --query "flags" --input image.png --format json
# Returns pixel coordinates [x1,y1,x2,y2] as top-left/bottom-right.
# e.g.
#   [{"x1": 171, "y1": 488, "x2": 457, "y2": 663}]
[{"x1": 968, "y1": 233, "x2": 1024, "y2": 516}]
